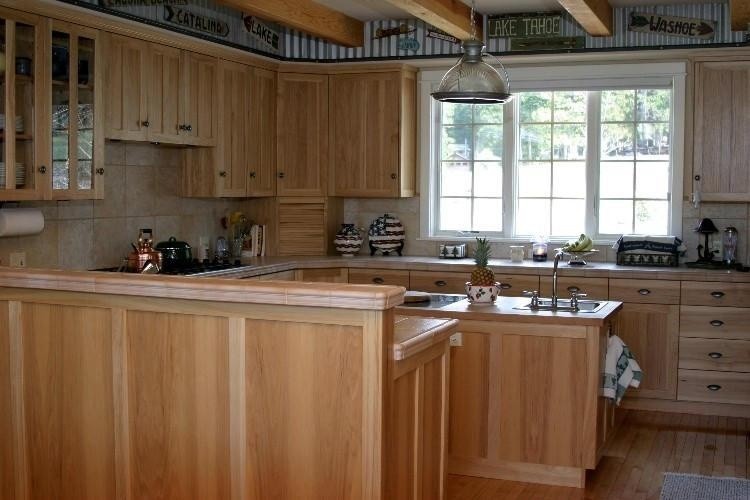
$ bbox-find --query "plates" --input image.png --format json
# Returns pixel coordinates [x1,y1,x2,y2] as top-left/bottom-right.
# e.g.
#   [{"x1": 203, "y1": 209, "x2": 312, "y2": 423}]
[{"x1": 467, "y1": 300, "x2": 496, "y2": 306}]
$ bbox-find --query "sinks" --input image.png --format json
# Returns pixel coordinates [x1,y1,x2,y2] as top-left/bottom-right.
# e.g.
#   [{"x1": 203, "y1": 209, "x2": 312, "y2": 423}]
[{"x1": 512, "y1": 300, "x2": 608, "y2": 313}]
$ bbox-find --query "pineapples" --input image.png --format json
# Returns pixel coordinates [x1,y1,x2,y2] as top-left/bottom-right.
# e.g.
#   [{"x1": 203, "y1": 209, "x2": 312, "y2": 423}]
[{"x1": 470, "y1": 235, "x2": 495, "y2": 287}]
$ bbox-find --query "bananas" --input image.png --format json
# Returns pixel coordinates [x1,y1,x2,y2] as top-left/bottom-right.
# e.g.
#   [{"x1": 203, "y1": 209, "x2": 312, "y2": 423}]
[{"x1": 564, "y1": 234, "x2": 593, "y2": 254}]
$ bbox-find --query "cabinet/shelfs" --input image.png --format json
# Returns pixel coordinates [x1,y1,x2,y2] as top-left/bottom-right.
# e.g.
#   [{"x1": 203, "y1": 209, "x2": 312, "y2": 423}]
[
  {"x1": 220, "y1": 47, "x2": 278, "y2": 200},
  {"x1": 0, "y1": 2, "x2": 103, "y2": 199},
  {"x1": 296, "y1": 268, "x2": 750, "y2": 419},
  {"x1": 104, "y1": 15, "x2": 219, "y2": 146},
  {"x1": 692, "y1": 46, "x2": 749, "y2": 203},
  {"x1": 329, "y1": 61, "x2": 420, "y2": 199},
  {"x1": 282, "y1": 61, "x2": 330, "y2": 203}
]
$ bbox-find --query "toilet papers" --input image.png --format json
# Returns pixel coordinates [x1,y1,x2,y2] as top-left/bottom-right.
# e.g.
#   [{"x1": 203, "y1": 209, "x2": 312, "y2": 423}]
[{"x1": 0, "y1": 208, "x2": 45, "y2": 238}]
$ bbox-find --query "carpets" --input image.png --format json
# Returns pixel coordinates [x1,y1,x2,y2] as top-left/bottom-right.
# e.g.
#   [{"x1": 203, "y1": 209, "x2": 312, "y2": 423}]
[{"x1": 659, "y1": 471, "x2": 750, "y2": 500}]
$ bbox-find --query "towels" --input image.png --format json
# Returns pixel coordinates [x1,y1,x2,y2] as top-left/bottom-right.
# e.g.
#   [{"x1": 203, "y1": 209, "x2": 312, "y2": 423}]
[{"x1": 612, "y1": 234, "x2": 687, "y2": 257}]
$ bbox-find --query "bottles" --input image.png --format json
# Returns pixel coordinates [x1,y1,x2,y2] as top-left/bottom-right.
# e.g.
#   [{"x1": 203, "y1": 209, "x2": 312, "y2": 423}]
[{"x1": 722, "y1": 223, "x2": 738, "y2": 263}]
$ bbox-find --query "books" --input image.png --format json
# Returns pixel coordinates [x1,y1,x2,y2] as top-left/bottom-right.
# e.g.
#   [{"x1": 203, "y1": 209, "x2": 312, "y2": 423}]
[{"x1": 233, "y1": 224, "x2": 266, "y2": 257}]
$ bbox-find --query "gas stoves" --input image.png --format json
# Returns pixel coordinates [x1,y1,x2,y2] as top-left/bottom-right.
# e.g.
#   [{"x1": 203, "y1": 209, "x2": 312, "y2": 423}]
[{"x1": 89, "y1": 257, "x2": 256, "y2": 277}]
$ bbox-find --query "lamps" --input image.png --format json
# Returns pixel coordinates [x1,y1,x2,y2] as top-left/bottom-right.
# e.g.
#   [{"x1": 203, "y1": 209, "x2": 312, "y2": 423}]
[{"x1": 428, "y1": 1, "x2": 514, "y2": 105}]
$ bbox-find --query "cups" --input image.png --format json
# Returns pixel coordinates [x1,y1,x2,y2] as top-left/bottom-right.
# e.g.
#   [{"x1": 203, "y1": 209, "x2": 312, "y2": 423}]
[
  {"x1": 532, "y1": 242, "x2": 548, "y2": 263},
  {"x1": 198, "y1": 235, "x2": 210, "y2": 263},
  {"x1": 623, "y1": 253, "x2": 670, "y2": 263},
  {"x1": 216, "y1": 239, "x2": 229, "y2": 259},
  {"x1": 228, "y1": 237, "x2": 243, "y2": 258},
  {"x1": 508, "y1": 245, "x2": 526, "y2": 264}
]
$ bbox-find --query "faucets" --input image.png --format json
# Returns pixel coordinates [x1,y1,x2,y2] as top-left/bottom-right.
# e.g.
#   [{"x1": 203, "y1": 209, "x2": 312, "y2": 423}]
[{"x1": 552, "y1": 252, "x2": 562, "y2": 308}]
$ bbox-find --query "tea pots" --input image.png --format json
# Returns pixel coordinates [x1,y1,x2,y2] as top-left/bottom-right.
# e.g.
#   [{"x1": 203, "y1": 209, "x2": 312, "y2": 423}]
[{"x1": 127, "y1": 228, "x2": 164, "y2": 274}]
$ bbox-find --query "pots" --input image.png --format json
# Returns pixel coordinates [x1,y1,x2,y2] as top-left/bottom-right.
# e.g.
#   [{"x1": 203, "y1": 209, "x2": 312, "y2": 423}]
[{"x1": 154, "y1": 237, "x2": 193, "y2": 268}]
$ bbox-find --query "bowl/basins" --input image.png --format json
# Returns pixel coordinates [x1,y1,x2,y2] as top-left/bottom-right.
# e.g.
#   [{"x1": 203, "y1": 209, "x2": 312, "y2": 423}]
[{"x1": 464, "y1": 281, "x2": 502, "y2": 302}]
